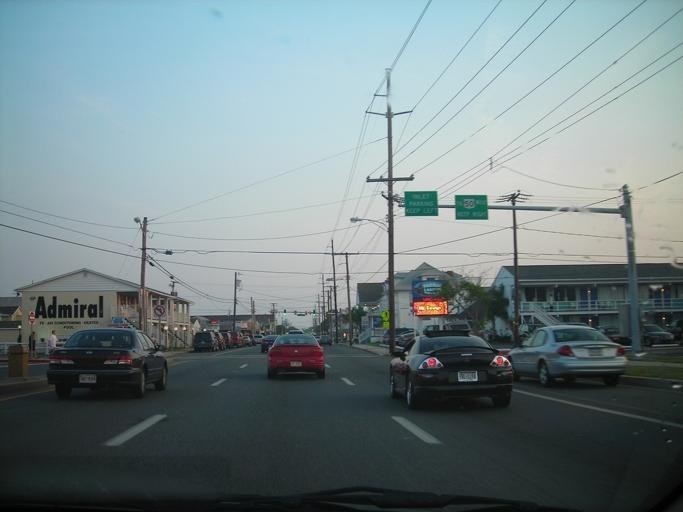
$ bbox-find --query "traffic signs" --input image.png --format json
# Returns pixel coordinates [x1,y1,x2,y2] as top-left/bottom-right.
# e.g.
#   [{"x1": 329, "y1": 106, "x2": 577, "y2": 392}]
[
  {"x1": 454, "y1": 195, "x2": 488, "y2": 220},
  {"x1": 405, "y1": 191, "x2": 438, "y2": 217}
]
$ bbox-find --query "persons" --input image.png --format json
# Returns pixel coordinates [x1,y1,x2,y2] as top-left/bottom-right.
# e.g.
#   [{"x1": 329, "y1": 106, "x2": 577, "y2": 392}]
[
  {"x1": 47, "y1": 329, "x2": 59, "y2": 354},
  {"x1": 28, "y1": 331, "x2": 40, "y2": 359}
]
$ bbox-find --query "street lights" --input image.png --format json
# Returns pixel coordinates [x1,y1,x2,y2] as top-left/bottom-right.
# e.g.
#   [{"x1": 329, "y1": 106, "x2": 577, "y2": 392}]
[{"x1": 348, "y1": 216, "x2": 394, "y2": 349}]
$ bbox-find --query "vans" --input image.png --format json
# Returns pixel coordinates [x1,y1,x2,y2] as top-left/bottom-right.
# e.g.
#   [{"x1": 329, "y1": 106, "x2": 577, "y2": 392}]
[
  {"x1": 253, "y1": 334, "x2": 264, "y2": 344},
  {"x1": 288, "y1": 330, "x2": 303, "y2": 335}
]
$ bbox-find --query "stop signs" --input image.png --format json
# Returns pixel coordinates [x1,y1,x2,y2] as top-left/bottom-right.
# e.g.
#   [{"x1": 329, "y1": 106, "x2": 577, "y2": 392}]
[{"x1": 27, "y1": 311, "x2": 36, "y2": 326}]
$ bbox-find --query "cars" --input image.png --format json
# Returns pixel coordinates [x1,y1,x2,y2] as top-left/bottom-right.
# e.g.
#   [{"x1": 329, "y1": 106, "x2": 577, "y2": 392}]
[
  {"x1": 261, "y1": 335, "x2": 280, "y2": 353},
  {"x1": 509, "y1": 326, "x2": 626, "y2": 387},
  {"x1": 314, "y1": 332, "x2": 331, "y2": 345},
  {"x1": 601, "y1": 317, "x2": 681, "y2": 347},
  {"x1": 390, "y1": 331, "x2": 513, "y2": 408},
  {"x1": 46, "y1": 329, "x2": 167, "y2": 398},
  {"x1": 267, "y1": 334, "x2": 325, "y2": 380}
]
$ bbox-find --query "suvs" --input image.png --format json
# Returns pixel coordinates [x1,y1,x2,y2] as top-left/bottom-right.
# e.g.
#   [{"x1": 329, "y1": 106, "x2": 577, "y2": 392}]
[
  {"x1": 194, "y1": 330, "x2": 256, "y2": 352},
  {"x1": 381, "y1": 328, "x2": 418, "y2": 354}
]
